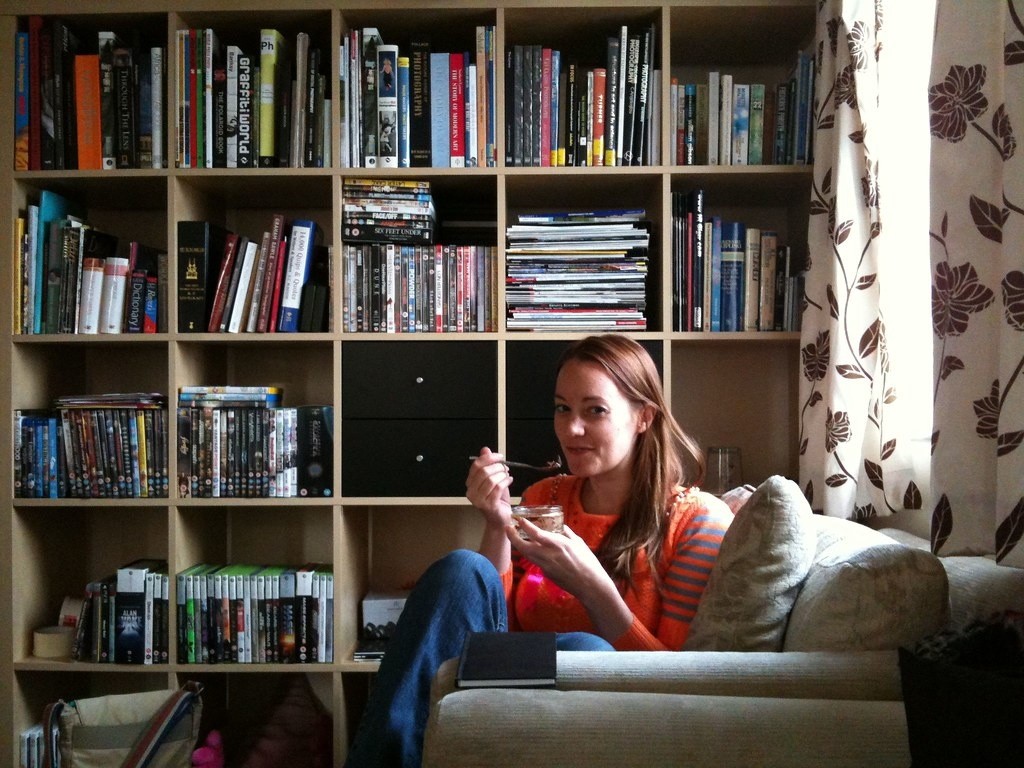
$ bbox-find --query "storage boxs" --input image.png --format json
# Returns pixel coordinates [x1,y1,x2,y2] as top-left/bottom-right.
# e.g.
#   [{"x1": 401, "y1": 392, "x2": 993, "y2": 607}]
[{"x1": 362, "y1": 589, "x2": 414, "y2": 649}]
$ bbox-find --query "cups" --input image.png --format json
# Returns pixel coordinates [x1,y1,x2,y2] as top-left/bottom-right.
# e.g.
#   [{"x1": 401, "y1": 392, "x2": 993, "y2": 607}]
[{"x1": 703, "y1": 445, "x2": 744, "y2": 494}]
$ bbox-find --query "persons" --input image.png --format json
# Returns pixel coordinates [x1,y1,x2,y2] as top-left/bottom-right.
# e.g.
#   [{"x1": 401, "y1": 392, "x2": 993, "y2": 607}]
[{"x1": 371, "y1": 335, "x2": 734, "y2": 768}]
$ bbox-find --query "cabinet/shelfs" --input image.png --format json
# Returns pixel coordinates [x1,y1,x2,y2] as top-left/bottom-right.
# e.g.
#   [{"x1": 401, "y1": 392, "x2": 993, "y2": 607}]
[{"x1": 0, "y1": 0, "x2": 819, "y2": 768}]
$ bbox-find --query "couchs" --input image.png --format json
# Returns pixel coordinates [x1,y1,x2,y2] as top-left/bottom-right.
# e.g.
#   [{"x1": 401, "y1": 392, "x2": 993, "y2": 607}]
[{"x1": 421, "y1": 484, "x2": 1024, "y2": 768}]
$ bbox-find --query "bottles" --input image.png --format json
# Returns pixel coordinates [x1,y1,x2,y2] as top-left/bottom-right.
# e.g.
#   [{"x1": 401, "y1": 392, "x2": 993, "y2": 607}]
[{"x1": 512, "y1": 504, "x2": 564, "y2": 539}]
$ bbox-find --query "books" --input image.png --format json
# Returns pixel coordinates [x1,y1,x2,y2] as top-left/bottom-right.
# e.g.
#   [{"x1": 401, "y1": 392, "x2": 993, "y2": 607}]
[
  {"x1": 19, "y1": 719, "x2": 61, "y2": 768},
  {"x1": 13, "y1": 386, "x2": 334, "y2": 664},
  {"x1": 8, "y1": 15, "x2": 818, "y2": 334},
  {"x1": 453, "y1": 630, "x2": 557, "y2": 688},
  {"x1": 353, "y1": 642, "x2": 385, "y2": 661}
]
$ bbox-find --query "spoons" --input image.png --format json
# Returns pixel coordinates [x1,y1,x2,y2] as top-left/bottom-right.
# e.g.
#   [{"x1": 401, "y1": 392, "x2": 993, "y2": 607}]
[{"x1": 469, "y1": 456, "x2": 559, "y2": 471}]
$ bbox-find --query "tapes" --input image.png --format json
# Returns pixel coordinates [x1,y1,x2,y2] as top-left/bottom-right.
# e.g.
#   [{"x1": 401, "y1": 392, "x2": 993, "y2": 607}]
[{"x1": 33, "y1": 625, "x2": 78, "y2": 658}]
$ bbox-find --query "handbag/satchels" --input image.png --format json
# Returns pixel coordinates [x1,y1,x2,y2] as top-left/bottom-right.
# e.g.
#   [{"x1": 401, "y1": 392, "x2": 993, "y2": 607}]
[{"x1": 43, "y1": 679, "x2": 205, "y2": 768}]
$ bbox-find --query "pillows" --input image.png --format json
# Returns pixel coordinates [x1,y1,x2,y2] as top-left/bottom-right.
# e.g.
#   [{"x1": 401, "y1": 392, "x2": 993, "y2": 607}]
[{"x1": 684, "y1": 475, "x2": 818, "y2": 653}]
